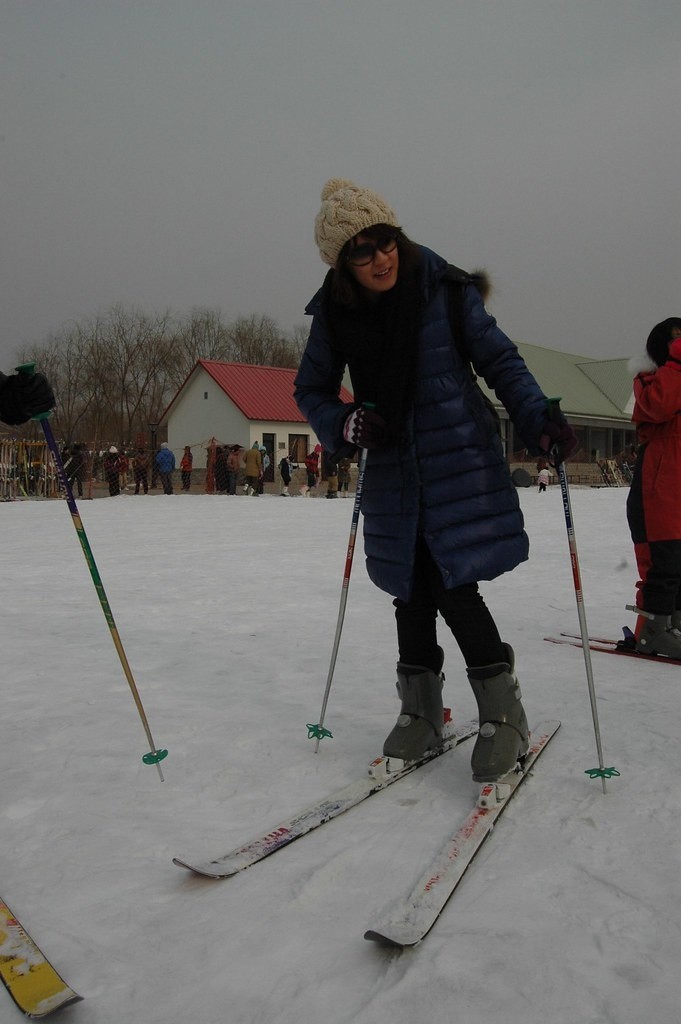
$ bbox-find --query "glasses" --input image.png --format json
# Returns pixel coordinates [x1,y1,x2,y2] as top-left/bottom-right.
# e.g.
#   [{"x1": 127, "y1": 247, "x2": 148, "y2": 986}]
[{"x1": 344, "y1": 235, "x2": 399, "y2": 267}]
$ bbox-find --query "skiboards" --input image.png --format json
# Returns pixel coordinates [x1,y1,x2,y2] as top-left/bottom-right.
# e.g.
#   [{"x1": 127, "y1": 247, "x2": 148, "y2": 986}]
[
  {"x1": 543, "y1": 622, "x2": 679, "y2": 664},
  {"x1": 0, "y1": 899, "x2": 84, "y2": 1023},
  {"x1": 171, "y1": 715, "x2": 564, "y2": 947}
]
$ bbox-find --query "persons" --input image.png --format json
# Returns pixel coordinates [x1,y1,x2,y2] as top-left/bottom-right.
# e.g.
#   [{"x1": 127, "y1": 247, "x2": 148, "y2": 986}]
[
  {"x1": 155, "y1": 441, "x2": 176, "y2": 496},
  {"x1": 181, "y1": 445, "x2": 194, "y2": 492},
  {"x1": 68, "y1": 445, "x2": 86, "y2": 498},
  {"x1": 325, "y1": 463, "x2": 338, "y2": 500},
  {"x1": 103, "y1": 446, "x2": 121, "y2": 497},
  {"x1": 538, "y1": 467, "x2": 555, "y2": 493},
  {"x1": 119, "y1": 452, "x2": 130, "y2": 491},
  {"x1": 536, "y1": 455, "x2": 556, "y2": 474},
  {"x1": 256, "y1": 446, "x2": 270, "y2": 494},
  {"x1": 0, "y1": 370, "x2": 56, "y2": 427},
  {"x1": 205, "y1": 442, "x2": 245, "y2": 496},
  {"x1": 60, "y1": 446, "x2": 69, "y2": 466},
  {"x1": 243, "y1": 440, "x2": 264, "y2": 496},
  {"x1": 626, "y1": 316, "x2": 681, "y2": 660},
  {"x1": 292, "y1": 176, "x2": 582, "y2": 784},
  {"x1": 280, "y1": 454, "x2": 299, "y2": 497},
  {"x1": 337, "y1": 457, "x2": 352, "y2": 497},
  {"x1": 305, "y1": 443, "x2": 323, "y2": 497},
  {"x1": 133, "y1": 447, "x2": 149, "y2": 495}
]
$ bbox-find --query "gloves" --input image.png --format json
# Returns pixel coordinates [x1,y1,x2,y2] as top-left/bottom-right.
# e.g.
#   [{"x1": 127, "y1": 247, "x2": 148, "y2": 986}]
[
  {"x1": 343, "y1": 407, "x2": 386, "y2": 449},
  {"x1": 541, "y1": 415, "x2": 578, "y2": 458},
  {"x1": 9, "y1": 373, "x2": 57, "y2": 421}
]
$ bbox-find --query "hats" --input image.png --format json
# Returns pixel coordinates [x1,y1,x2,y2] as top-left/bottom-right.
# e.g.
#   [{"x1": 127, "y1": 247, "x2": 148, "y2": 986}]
[
  {"x1": 182, "y1": 446, "x2": 190, "y2": 450},
  {"x1": 109, "y1": 446, "x2": 118, "y2": 454},
  {"x1": 313, "y1": 175, "x2": 401, "y2": 269}
]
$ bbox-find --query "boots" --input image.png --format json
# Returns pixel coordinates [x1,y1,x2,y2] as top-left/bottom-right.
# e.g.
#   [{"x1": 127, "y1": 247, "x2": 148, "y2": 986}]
[
  {"x1": 382, "y1": 645, "x2": 446, "y2": 760},
  {"x1": 463, "y1": 641, "x2": 529, "y2": 782},
  {"x1": 243, "y1": 483, "x2": 349, "y2": 498},
  {"x1": 625, "y1": 605, "x2": 681, "y2": 660}
]
